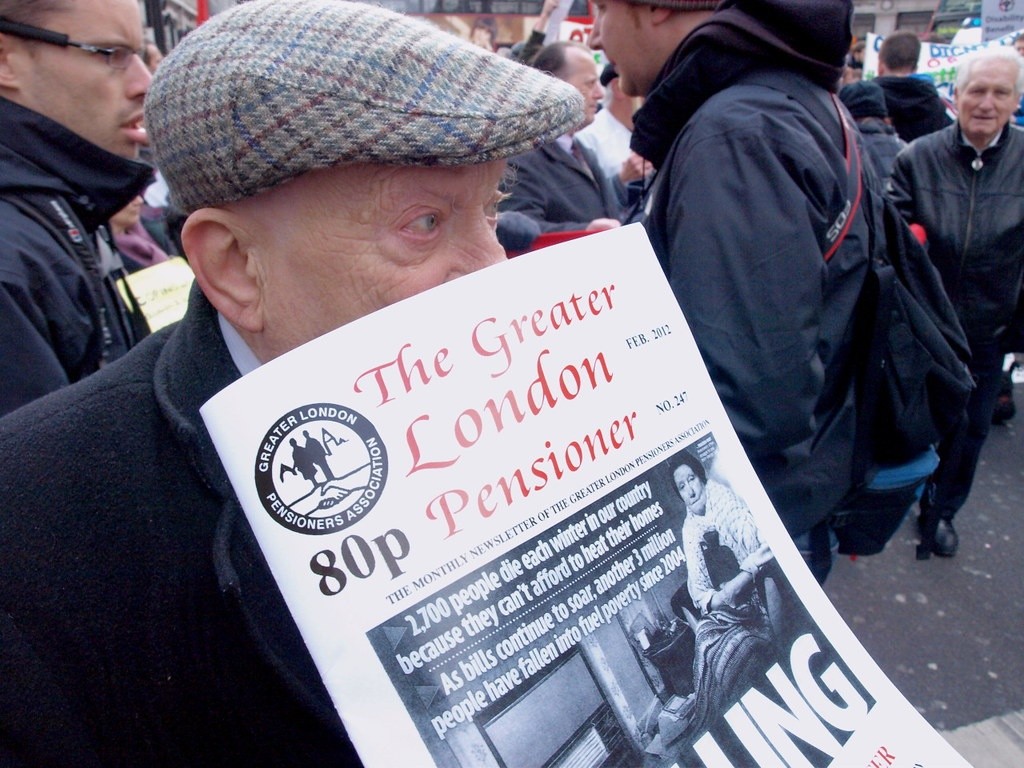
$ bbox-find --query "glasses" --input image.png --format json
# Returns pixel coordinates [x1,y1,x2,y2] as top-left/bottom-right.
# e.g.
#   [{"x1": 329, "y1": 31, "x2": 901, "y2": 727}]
[{"x1": 0, "y1": 19, "x2": 147, "y2": 71}]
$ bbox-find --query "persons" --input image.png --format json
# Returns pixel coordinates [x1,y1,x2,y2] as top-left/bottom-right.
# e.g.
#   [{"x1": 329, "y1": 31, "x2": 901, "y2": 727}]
[
  {"x1": 0, "y1": 0, "x2": 1024, "y2": 590},
  {"x1": 0, "y1": 0, "x2": 583, "y2": 768},
  {"x1": 665, "y1": 452, "x2": 779, "y2": 614}
]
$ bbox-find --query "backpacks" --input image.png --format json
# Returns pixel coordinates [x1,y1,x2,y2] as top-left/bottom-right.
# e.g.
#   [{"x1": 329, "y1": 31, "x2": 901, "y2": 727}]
[{"x1": 819, "y1": 93, "x2": 976, "y2": 556}]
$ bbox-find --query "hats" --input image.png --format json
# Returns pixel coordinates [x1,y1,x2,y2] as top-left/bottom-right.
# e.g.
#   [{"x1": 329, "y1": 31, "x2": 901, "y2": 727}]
[
  {"x1": 599, "y1": 63, "x2": 619, "y2": 87},
  {"x1": 145, "y1": 0, "x2": 585, "y2": 214},
  {"x1": 839, "y1": 79, "x2": 890, "y2": 118}
]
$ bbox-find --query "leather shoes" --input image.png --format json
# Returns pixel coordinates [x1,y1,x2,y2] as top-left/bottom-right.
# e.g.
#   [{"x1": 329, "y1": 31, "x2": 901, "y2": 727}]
[{"x1": 920, "y1": 506, "x2": 958, "y2": 556}]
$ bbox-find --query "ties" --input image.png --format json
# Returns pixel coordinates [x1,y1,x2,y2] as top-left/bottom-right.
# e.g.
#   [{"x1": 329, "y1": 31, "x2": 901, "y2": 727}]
[{"x1": 571, "y1": 142, "x2": 600, "y2": 189}]
